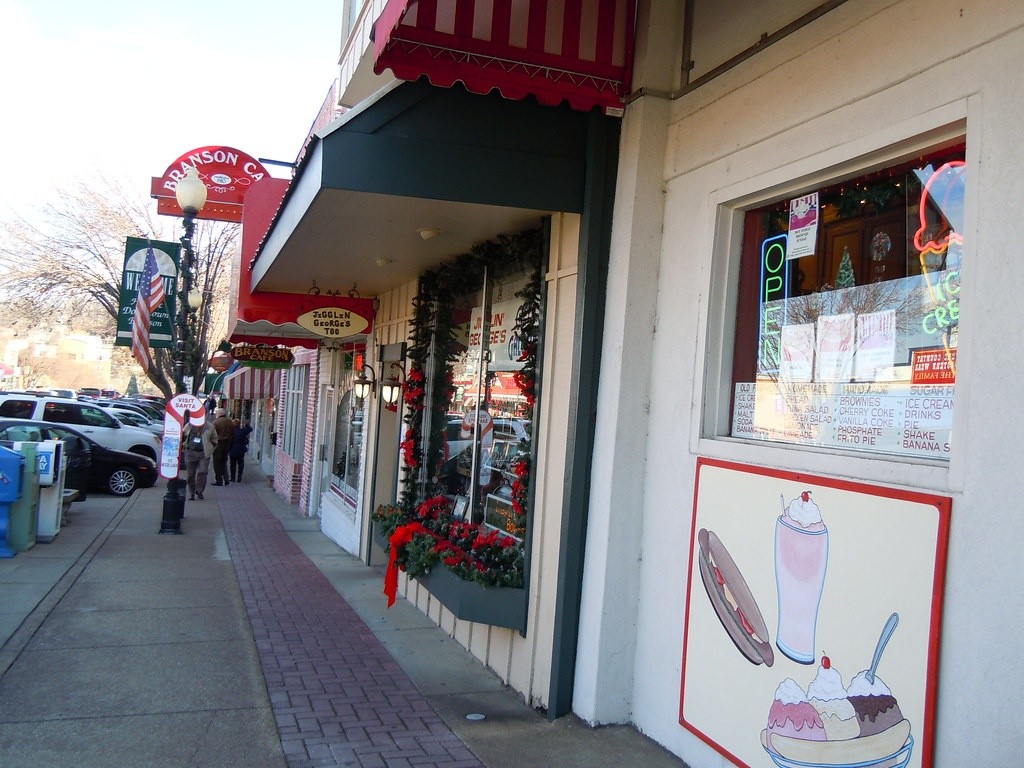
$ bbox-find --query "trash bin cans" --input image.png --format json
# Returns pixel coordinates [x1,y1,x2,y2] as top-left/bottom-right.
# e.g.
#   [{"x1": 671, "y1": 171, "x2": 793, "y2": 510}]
[{"x1": 1, "y1": 437, "x2": 67, "y2": 559}]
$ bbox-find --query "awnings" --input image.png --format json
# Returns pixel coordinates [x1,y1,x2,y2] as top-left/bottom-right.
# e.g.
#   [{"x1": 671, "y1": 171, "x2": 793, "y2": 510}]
[
  {"x1": 370, "y1": 0, "x2": 638, "y2": 118},
  {"x1": 223, "y1": 369, "x2": 279, "y2": 399},
  {"x1": 225, "y1": 178, "x2": 377, "y2": 350},
  {"x1": 246, "y1": 76, "x2": 583, "y2": 300},
  {"x1": 209, "y1": 362, "x2": 241, "y2": 396}
]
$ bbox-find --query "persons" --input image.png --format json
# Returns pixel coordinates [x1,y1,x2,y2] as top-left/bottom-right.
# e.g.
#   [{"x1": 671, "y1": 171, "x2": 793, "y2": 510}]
[
  {"x1": 181, "y1": 420, "x2": 218, "y2": 500},
  {"x1": 211, "y1": 408, "x2": 253, "y2": 486},
  {"x1": 203, "y1": 396, "x2": 222, "y2": 417}
]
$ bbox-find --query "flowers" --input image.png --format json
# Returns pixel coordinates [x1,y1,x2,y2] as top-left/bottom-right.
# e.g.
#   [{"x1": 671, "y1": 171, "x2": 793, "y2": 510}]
[{"x1": 370, "y1": 503, "x2": 523, "y2": 589}]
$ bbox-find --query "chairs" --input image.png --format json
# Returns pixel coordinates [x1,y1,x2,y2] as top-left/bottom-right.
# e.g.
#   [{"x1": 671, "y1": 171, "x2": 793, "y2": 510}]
[{"x1": 28, "y1": 431, "x2": 38, "y2": 442}]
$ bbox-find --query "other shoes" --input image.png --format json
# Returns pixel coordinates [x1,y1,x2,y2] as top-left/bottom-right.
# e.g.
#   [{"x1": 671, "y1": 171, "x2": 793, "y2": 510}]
[
  {"x1": 188, "y1": 490, "x2": 204, "y2": 500},
  {"x1": 229, "y1": 479, "x2": 235, "y2": 482},
  {"x1": 210, "y1": 478, "x2": 230, "y2": 486}
]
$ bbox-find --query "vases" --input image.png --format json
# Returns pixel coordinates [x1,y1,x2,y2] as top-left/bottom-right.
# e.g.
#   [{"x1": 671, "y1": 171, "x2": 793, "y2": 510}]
[{"x1": 374, "y1": 520, "x2": 526, "y2": 632}]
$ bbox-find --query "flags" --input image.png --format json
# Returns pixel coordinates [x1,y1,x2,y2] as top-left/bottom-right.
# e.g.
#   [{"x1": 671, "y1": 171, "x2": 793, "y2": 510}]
[{"x1": 131, "y1": 243, "x2": 165, "y2": 374}]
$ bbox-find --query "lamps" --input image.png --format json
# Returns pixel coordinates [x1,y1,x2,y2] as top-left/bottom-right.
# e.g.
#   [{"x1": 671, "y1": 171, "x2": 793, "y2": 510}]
[
  {"x1": 378, "y1": 362, "x2": 407, "y2": 407},
  {"x1": 354, "y1": 364, "x2": 377, "y2": 400}
]
